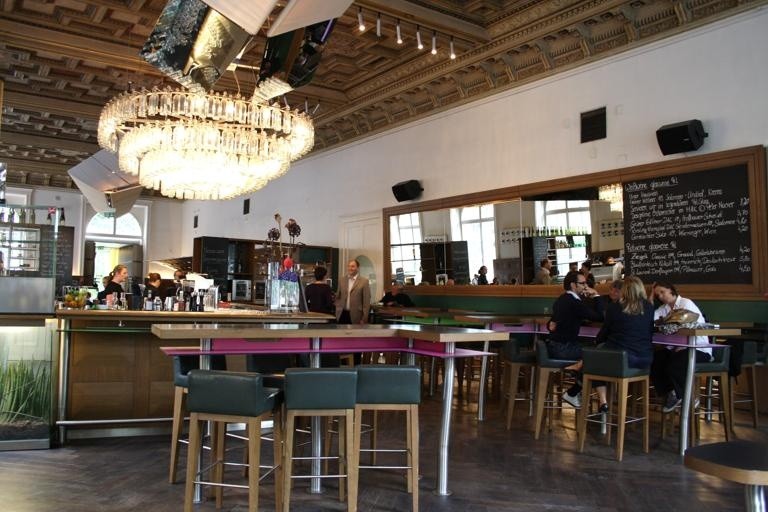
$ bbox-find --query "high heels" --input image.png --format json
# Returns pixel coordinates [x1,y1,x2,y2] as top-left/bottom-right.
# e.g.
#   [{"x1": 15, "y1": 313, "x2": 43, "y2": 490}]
[
  {"x1": 560, "y1": 368, "x2": 577, "y2": 377},
  {"x1": 598, "y1": 403, "x2": 609, "y2": 415}
]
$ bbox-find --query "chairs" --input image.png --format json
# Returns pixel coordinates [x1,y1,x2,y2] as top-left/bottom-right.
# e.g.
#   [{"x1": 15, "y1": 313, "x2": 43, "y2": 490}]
[
  {"x1": 282, "y1": 367, "x2": 357, "y2": 512},
  {"x1": 535, "y1": 340, "x2": 598, "y2": 440},
  {"x1": 183, "y1": 369, "x2": 283, "y2": 512},
  {"x1": 729, "y1": 341, "x2": 759, "y2": 428},
  {"x1": 455, "y1": 341, "x2": 499, "y2": 392},
  {"x1": 297, "y1": 352, "x2": 377, "y2": 475},
  {"x1": 499, "y1": 335, "x2": 554, "y2": 432},
  {"x1": 240, "y1": 353, "x2": 297, "y2": 478},
  {"x1": 577, "y1": 347, "x2": 651, "y2": 462},
  {"x1": 660, "y1": 345, "x2": 731, "y2": 448},
  {"x1": 354, "y1": 364, "x2": 422, "y2": 511},
  {"x1": 169, "y1": 354, "x2": 249, "y2": 484}
]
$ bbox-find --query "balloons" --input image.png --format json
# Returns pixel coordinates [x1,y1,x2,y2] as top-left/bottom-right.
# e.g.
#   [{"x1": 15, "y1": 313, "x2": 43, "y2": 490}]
[{"x1": 284, "y1": 258, "x2": 293, "y2": 269}]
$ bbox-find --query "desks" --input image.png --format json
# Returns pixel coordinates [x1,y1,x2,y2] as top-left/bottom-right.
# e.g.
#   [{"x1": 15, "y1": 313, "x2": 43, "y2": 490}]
[{"x1": 150, "y1": 323, "x2": 510, "y2": 505}]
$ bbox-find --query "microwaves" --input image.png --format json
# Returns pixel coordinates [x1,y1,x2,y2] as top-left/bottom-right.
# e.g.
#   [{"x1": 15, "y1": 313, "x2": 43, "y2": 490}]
[{"x1": 225, "y1": 279, "x2": 253, "y2": 303}]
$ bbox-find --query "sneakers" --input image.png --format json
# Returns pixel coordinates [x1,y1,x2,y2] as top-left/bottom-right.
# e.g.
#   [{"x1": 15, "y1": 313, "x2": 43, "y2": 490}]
[
  {"x1": 694, "y1": 398, "x2": 700, "y2": 409},
  {"x1": 561, "y1": 391, "x2": 583, "y2": 409},
  {"x1": 662, "y1": 392, "x2": 682, "y2": 413}
]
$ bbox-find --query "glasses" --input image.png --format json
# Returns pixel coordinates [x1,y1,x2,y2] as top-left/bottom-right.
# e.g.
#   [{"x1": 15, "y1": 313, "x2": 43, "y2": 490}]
[{"x1": 572, "y1": 281, "x2": 587, "y2": 286}]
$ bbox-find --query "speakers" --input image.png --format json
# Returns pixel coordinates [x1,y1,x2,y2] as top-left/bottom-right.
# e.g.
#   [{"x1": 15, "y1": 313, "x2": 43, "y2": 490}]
[
  {"x1": 391, "y1": 179, "x2": 424, "y2": 202},
  {"x1": 655, "y1": 119, "x2": 707, "y2": 155}
]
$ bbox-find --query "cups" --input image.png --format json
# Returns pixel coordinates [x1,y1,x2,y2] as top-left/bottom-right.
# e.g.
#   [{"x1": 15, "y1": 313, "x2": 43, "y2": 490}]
[{"x1": 203, "y1": 294, "x2": 216, "y2": 313}]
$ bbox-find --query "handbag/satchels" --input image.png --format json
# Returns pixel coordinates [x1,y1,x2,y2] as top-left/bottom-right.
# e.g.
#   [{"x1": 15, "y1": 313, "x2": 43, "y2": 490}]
[{"x1": 655, "y1": 308, "x2": 699, "y2": 326}]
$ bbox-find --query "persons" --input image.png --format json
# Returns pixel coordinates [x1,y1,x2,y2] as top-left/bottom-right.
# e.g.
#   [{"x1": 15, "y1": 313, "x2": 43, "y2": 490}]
[
  {"x1": 299, "y1": 267, "x2": 333, "y2": 315},
  {"x1": 547, "y1": 272, "x2": 605, "y2": 409},
  {"x1": 102, "y1": 276, "x2": 111, "y2": 289},
  {"x1": 649, "y1": 280, "x2": 713, "y2": 413},
  {"x1": 569, "y1": 262, "x2": 578, "y2": 273},
  {"x1": 335, "y1": 260, "x2": 370, "y2": 325},
  {"x1": 601, "y1": 279, "x2": 620, "y2": 304},
  {"x1": 535, "y1": 259, "x2": 553, "y2": 285},
  {"x1": 579, "y1": 262, "x2": 595, "y2": 288},
  {"x1": 478, "y1": 265, "x2": 488, "y2": 285},
  {"x1": 583, "y1": 270, "x2": 658, "y2": 415},
  {"x1": 379, "y1": 280, "x2": 415, "y2": 308},
  {"x1": 143, "y1": 272, "x2": 161, "y2": 300},
  {"x1": 98, "y1": 265, "x2": 128, "y2": 306}
]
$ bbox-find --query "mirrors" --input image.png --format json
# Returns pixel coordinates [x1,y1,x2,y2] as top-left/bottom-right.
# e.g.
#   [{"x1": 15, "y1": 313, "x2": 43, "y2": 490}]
[
  {"x1": 388, "y1": 195, "x2": 523, "y2": 287},
  {"x1": 519, "y1": 182, "x2": 625, "y2": 286}
]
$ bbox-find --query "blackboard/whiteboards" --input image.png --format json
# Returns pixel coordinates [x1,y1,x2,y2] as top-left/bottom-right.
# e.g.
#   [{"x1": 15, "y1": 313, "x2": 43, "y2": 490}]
[
  {"x1": 41, "y1": 225, "x2": 75, "y2": 280},
  {"x1": 621, "y1": 144, "x2": 768, "y2": 301}
]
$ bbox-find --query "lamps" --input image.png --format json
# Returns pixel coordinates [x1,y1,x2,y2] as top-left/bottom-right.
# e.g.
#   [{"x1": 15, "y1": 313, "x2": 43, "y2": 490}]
[
  {"x1": 97, "y1": 85, "x2": 316, "y2": 202},
  {"x1": 598, "y1": 183, "x2": 623, "y2": 218}
]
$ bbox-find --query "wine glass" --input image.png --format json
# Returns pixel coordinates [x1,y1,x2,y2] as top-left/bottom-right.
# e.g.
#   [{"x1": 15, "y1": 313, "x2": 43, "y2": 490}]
[{"x1": 111, "y1": 291, "x2": 126, "y2": 310}]
